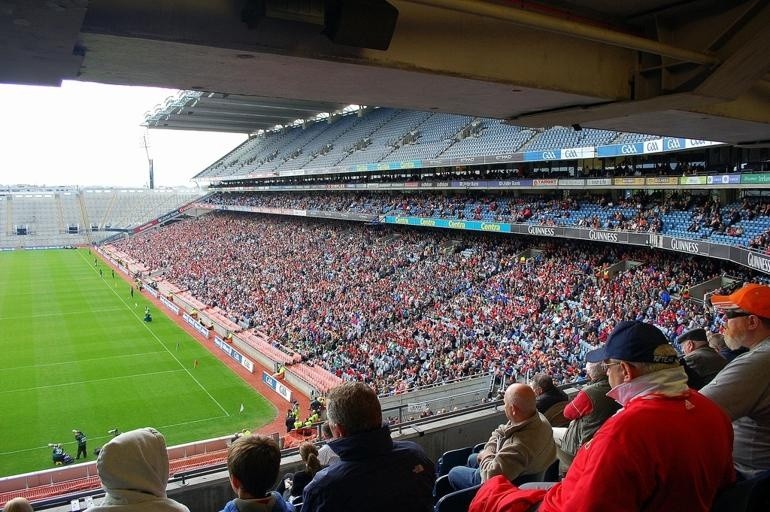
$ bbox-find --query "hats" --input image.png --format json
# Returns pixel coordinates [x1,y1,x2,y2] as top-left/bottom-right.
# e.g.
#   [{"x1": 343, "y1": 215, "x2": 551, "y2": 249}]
[
  {"x1": 711, "y1": 283, "x2": 770, "y2": 319},
  {"x1": 586, "y1": 321, "x2": 676, "y2": 363},
  {"x1": 677, "y1": 329, "x2": 707, "y2": 344}
]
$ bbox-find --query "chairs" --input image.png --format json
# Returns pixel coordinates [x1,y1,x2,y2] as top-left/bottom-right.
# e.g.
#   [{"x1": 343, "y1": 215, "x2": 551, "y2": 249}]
[
  {"x1": 188, "y1": 105, "x2": 769, "y2": 250},
  {"x1": 286, "y1": 442, "x2": 591, "y2": 510}
]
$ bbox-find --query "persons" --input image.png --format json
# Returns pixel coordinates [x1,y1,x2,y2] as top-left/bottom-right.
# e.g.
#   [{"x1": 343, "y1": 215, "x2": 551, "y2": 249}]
[
  {"x1": 83, "y1": 158, "x2": 770, "y2": 512},
  {"x1": 72, "y1": 429, "x2": 87, "y2": 459},
  {"x1": 2, "y1": 496, "x2": 35, "y2": 511},
  {"x1": 52, "y1": 442, "x2": 66, "y2": 464}
]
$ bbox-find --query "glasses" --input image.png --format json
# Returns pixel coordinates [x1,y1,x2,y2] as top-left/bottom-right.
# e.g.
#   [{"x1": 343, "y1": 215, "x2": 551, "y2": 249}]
[{"x1": 725, "y1": 311, "x2": 750, "y2": 319}]
